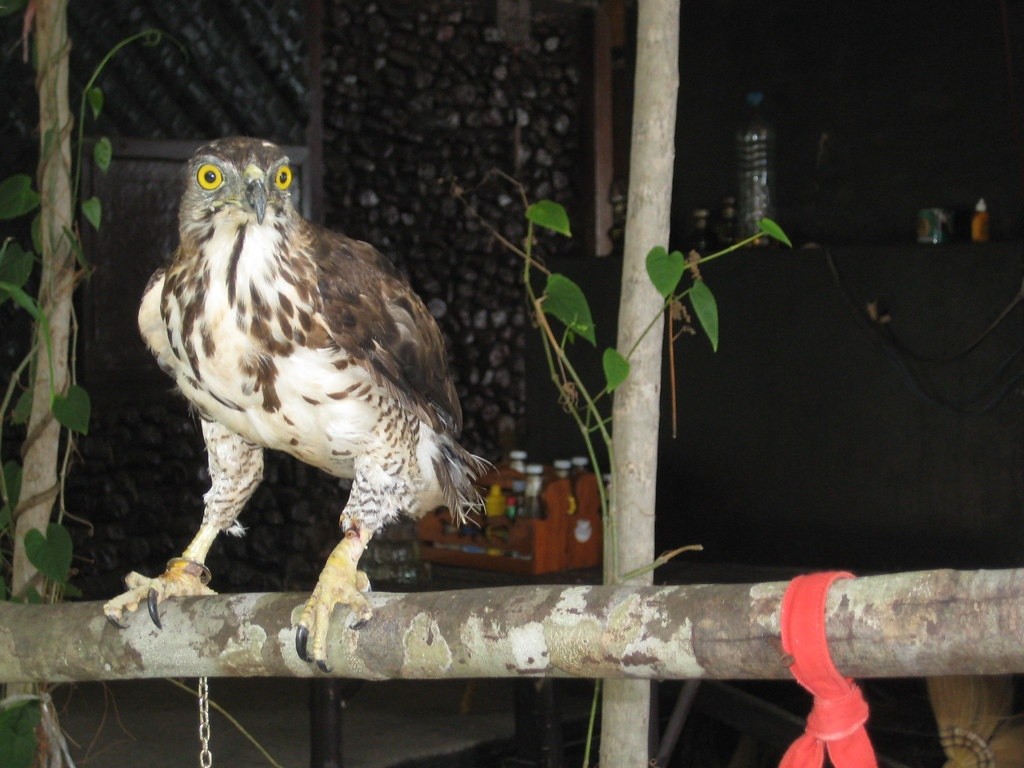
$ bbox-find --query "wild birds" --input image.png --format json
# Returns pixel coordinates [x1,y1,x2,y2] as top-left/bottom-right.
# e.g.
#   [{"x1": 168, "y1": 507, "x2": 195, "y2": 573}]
[{"x1": 101, "y1": 136, "x2": 487, "y2": 673}]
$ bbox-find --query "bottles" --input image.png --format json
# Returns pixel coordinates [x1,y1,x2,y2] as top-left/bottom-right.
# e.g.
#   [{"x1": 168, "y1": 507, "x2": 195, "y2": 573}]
[
  {"x1": 970, "y1": 195, "x2": 993, "y2": 244},
  {"x1": 432, "y1": 451, "x2": 589, "y2": 559}
]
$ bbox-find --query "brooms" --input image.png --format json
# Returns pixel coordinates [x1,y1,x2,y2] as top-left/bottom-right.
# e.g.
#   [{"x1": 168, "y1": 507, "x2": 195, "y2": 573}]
[{"x1": 929, "y1": 675, "x2": 1024, "y2": 768}]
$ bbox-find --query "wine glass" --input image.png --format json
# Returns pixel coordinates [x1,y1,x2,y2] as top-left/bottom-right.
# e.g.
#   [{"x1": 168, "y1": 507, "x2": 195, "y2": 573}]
[{"x1": 688, "y1": 196, "x2": 738, "y2": 254}]
